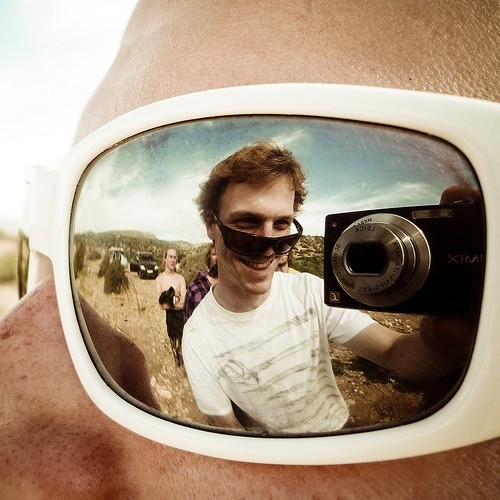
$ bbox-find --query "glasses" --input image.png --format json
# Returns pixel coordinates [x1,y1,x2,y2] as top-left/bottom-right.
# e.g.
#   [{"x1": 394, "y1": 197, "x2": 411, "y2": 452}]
[
  {"x1": 210, "y1": 213, "x2": 304, "y2": 256},
  {"x1": 11, "y1": 81, "x2": 499, "y2": 468}
]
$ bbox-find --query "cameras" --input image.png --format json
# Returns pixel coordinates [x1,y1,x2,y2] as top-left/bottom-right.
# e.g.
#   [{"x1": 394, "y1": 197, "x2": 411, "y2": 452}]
[{"x1": 322, "y1": 202, "x2": 486, "y2": 319}]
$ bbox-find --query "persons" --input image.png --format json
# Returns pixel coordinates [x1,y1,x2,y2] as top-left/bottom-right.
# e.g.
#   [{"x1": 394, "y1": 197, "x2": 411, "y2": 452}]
[
  {"x1": 180, "y1": 140, "x2": 488, "y2": 434},
  {"x1": 272, "y1": 249, "x2": 301, "y2": 274},
  {"x1": 183, "y1": 244, "x2": 218, "y2": 322},
  {"x1": 156, "y1": 248, "x2": 186, "y2": 366},
  {"x1": 0, "y1": 0, "x2": 500, "y2": 500}
]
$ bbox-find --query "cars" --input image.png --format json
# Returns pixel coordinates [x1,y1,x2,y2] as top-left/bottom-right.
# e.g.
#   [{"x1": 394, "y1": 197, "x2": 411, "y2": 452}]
[{"x1": 130, "y1": 251, "x2": 159, "y2": 279}]
